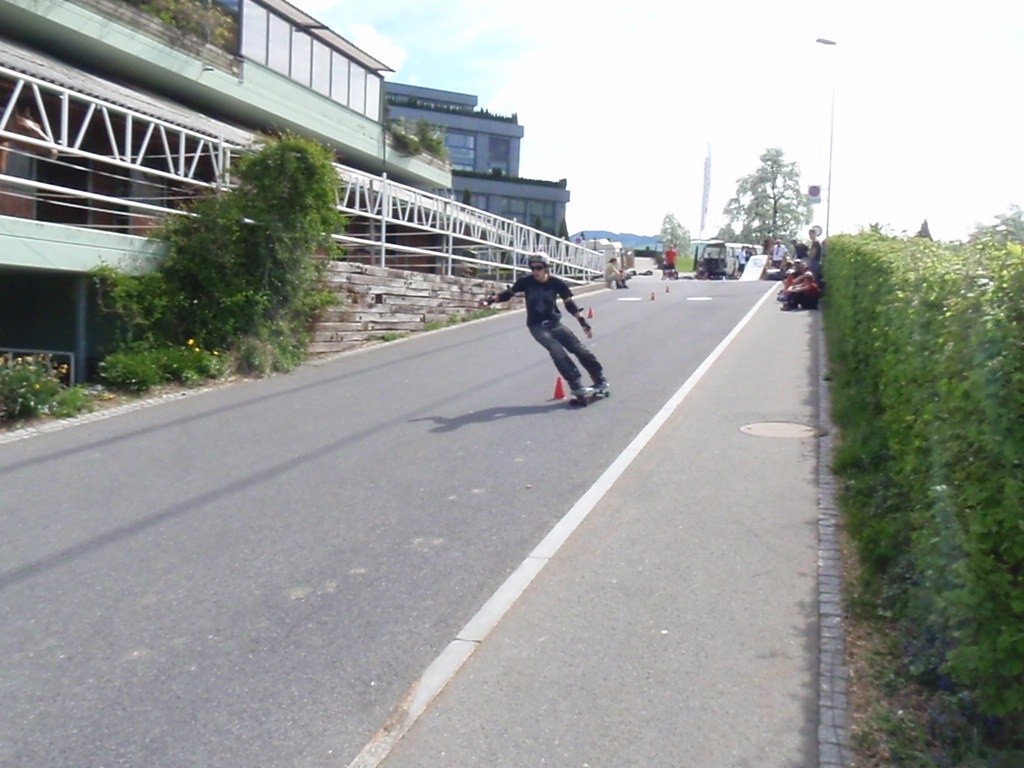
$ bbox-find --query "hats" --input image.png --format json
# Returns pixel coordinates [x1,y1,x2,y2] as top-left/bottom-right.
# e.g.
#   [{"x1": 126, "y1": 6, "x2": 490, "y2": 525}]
[{"x1": 804, "y1": 271, "x2": 813, "y2": 278}]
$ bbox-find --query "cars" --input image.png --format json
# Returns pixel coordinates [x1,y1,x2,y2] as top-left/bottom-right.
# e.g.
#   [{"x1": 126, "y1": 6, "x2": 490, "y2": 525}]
[{"x1": 692, "y1": 240, "x2": 739, "y2": 280}]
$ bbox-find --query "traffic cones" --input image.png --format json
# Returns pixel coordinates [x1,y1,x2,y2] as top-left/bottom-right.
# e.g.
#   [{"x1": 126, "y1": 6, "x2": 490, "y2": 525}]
[
  {"x1": 665, "y1": 285, "x2": 669, "y2": 293},
  {"x1": 587, "y1": 308, "x2": 594, "y2": 318},
  {"x1": 652, "y1": 292, "x2": 656, "y2": 300},
  {"x1": 554, "y1": 377, "x2": 566, "y2": 399}
]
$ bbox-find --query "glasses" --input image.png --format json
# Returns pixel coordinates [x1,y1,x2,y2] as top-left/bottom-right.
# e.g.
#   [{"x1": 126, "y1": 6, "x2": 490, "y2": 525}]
[{"x1": 530, "y1": 265, "x2": 546, "y2": 270}]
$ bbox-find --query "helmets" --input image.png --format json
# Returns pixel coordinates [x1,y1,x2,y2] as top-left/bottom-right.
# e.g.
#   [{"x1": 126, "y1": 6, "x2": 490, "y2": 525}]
[{"x1": 529, "y1": 252, "x2": 554, "y2": 267}]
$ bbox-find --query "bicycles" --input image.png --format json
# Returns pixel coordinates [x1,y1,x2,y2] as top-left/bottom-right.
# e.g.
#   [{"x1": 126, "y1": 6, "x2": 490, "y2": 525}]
[{"x1": 661, "y1": 262, "x2": 679, "y2": 281}]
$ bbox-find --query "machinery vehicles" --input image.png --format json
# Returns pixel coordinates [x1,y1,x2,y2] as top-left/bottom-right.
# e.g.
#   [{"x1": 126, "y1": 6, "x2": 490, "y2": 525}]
[{"x1": 699, "y1": 154, "x2": 711, "y2": 239}]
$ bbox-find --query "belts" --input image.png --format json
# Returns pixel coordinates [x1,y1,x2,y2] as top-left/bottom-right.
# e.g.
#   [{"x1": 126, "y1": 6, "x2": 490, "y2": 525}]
[{"x1": 529, "y1": 320, "x2": 555, "y2": 327}]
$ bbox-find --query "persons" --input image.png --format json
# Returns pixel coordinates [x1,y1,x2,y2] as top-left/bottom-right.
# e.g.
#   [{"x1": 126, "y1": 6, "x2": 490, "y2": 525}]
[
  {"x1": 739, "y1": 245, "x2": 753, "y2": 275},
  {"x1": 479, "y1": 252, "x2": 610, "y2": 396},
  {"x1": 663, "y1": 244, "x2": 679, "y2": 279},
  {"x1": 603, "y1": 257, "x2": 628, "y2": 288},
  {"x1": 772, "y1": 229, "x2": 822, "y2": 312}
]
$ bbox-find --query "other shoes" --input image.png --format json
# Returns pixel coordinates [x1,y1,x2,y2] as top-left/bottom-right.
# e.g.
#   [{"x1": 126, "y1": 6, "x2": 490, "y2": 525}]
[
  {"x1": 571, "y1": 385, "x2": 593, "y2": 395},
  {"x1": 778, "y1": 295, "x2": 791, "y2": 301},
  {"x1": 617, "y1": 284, "x2": 628, "y2": 288},
  {"x1": 781, "y1": 302, "x2": 798, "y2": 311},
  {"x1": 594, "y1": 380, "x2": 609, "y2": 387}
]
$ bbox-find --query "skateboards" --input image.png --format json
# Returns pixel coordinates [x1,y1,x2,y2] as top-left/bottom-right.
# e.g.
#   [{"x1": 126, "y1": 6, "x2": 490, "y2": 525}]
[{"x1": 567, "y1": 380, "x2": 612, "y2": 407}]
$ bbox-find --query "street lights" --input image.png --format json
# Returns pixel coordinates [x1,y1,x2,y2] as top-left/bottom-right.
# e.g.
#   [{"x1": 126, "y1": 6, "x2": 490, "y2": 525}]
[{"x1": 815, "y1": 36, "x2": 839, "y2": 238}]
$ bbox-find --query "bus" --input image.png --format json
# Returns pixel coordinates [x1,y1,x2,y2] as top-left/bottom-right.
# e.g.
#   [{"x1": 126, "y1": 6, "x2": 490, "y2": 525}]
[{"x1": 726, "y1": 243, "x2": 764, "y2": 271}]
